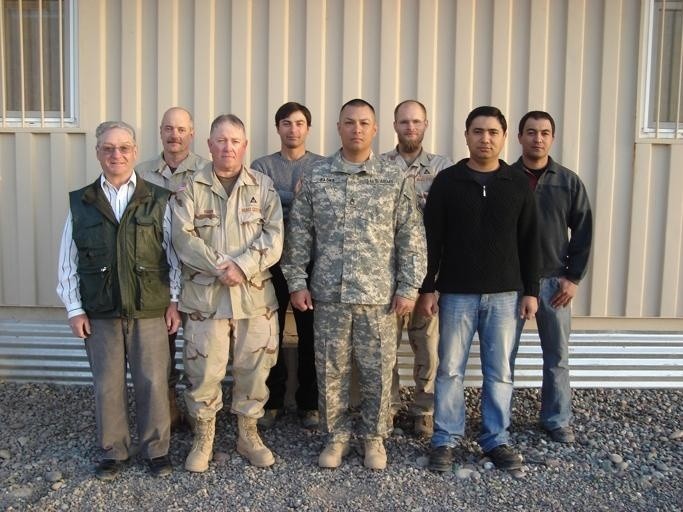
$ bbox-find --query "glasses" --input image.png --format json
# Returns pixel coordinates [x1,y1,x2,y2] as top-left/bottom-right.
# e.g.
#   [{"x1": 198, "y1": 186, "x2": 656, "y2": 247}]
[{"x1": 96, "y1": 142, "x2": 137, "y2": 155}]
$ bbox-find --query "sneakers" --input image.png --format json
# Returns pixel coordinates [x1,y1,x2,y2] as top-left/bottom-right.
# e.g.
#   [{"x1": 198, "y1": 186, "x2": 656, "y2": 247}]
[
  {"x1": 415, "y1": 413, "x2": 434, "y2": 437},
  {"x1": 358, "y1": 438, "x2": 387, "y2": 469},
  {"x1": 482, "y1": 444, "x2": 522, "y2": 468},
  {"x1": 257, "y1": 409, "x2": 284, "y2": 428},
  {"x1": 428, "y1": 445, "x2": 452, "y2": 472},
  {"x1": 96, "y1": 456, "x2": 131, "y2": 481},
  {"x1": 298, "y1": 406, "x2": 319, "y2": 427},
  {"x1": 318, "y1": 441, "x2": 350, "y2": 467},
  {"x1": 540, "y1": 422, "x2": 575, "y2": 444},
  {"x1": 145, "y1": 455, "x2": 172, "y2": 478}
]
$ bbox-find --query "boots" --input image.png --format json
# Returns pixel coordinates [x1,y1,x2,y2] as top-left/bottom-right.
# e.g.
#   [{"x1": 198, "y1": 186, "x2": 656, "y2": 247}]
[
  {"x1": 168, "y1": 387, "x2": 180, "y2": 430},
  {"x1": 184, "y1": 417, "x2": 216, "y2": 473},
  {"x1": 235, "y1": 413, "x2": 276, "y2": 467}
]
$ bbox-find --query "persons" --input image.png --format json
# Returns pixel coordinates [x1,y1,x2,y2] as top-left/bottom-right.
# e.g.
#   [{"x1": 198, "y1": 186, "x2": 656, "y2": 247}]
[
  {"x1": 509, "y1": 110, "x2": 592, "y2": 442},
  {"x1": 250, "y1": 102, "x2": 325, "y2": 427},
  {"x1": 415, "y1": 105, "x2": 540, "y2": 471},
  {"x1": 378, "y1": 100, "x2": 453, "y2": 437},
  {"x1": 135, "y1": 106, "x2": 212, "y2": 433},
  {"x1": 56, "y1": 121, "x2": 180, "y2": 481},
  {"x1": 278, "y1": 99, "x2": 427, "y2": 470},
  {"x1": 170, "y1": 113, "x2": 284, "y2": 472}
]
